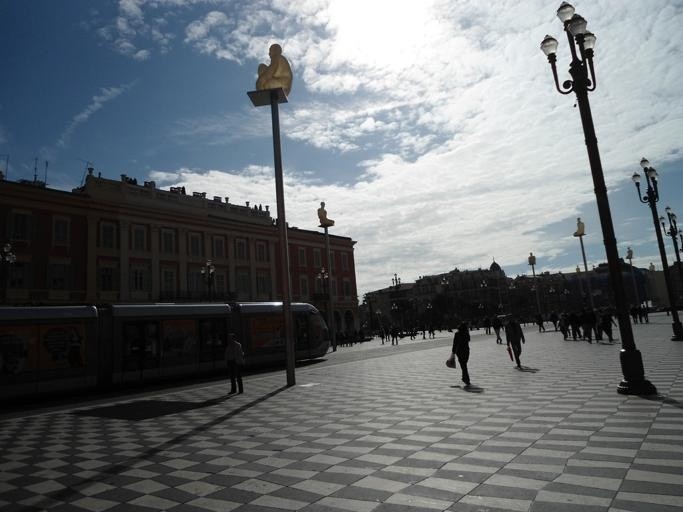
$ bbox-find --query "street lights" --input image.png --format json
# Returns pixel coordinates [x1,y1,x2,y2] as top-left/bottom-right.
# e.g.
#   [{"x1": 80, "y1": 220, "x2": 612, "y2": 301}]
[
  {"x1": 201, "y1": 260, "x2": 216, "y2": 302},
  {"x1": 441, "y1": 277, "x2": 449, "y2": 297},
  {"x1": 509, "y1": 282, "x2": 516, "y2": 290},
  {"x1": 540, "y1": 1, "x2": 656, "y2": 394},
  {"x1": 392, "y1": 273, "x2": 404, "y2": 338},
  {"x1": 478, "y1": 280, "x2": 487, "y2": 309},
  {"x1": 0, "y1": 240, "x2": 16, "y2": 265},
  {"x1": 317, "y1": 266, "x2": 328, "y2": 313},
  {"x1": 632, "y1": 157, "x2": 683, "y2": 341},
  {"x1": 531, "y1": 283, "x2": 570, "y2": 295}
]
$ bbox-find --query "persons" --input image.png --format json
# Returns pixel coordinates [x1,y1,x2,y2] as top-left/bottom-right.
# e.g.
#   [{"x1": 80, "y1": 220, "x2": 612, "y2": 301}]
[
  {"x1": 445, "y1": 322, "x2": 471, "y2": 387},
  {"x1": 223, "y1": 332, "x2": 244, "y2": 394},
  {"x1": 337, "y1": 316, "x2": 483, "y2": 348},
  {"x1": 483, "y1": 304, "x2": 649, "y2": 369}
]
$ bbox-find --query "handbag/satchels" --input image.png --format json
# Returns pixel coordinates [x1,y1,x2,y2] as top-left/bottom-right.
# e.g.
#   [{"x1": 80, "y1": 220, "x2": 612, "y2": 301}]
[
  {"x1": 445, "y1": 352, "x2": 456, "y2": 369},
  {"x1": 507, "y1": 345, "x2": 514, "y2": 362}
]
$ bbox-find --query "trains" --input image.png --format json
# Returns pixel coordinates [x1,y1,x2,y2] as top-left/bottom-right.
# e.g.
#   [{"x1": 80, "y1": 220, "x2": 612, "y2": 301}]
[{"x1": 0, "y1": 302, "x2": 329, "y2": 398}]
